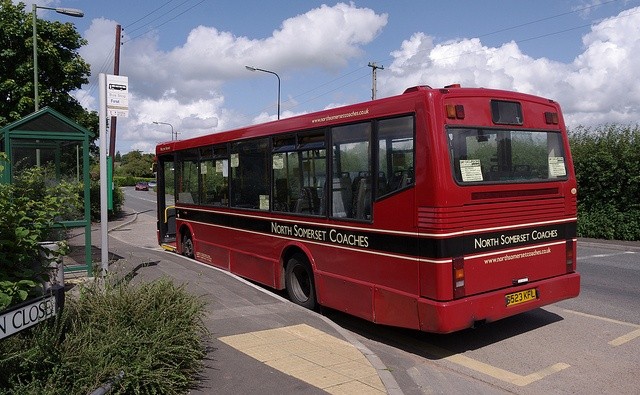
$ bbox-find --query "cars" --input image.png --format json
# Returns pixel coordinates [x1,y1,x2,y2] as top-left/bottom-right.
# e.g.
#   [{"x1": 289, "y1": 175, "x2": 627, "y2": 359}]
[
  {"x1": 135, "y1": 182, "x2": 149, "y2": 191},
  {"x1": 148, "y1": 180, "x2": 157, "y2": 187}
]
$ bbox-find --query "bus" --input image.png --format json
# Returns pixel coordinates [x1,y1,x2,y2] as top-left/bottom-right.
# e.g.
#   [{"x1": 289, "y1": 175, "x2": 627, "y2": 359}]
[{"x1": 156, "y1": 85, "x2": 580, "y2": 334}]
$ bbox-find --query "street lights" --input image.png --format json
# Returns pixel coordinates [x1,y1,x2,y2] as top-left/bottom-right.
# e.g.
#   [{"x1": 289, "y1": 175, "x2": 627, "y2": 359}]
[
  {"x1": 245, "y1": 66, "x2": 280, "y2": 120},
  {"x1": 32, "y1": 2, "x2": 84, "y2": 171},
  {"x1": 153, "y1": 121, "x2": 173, "y2": 141}
]
